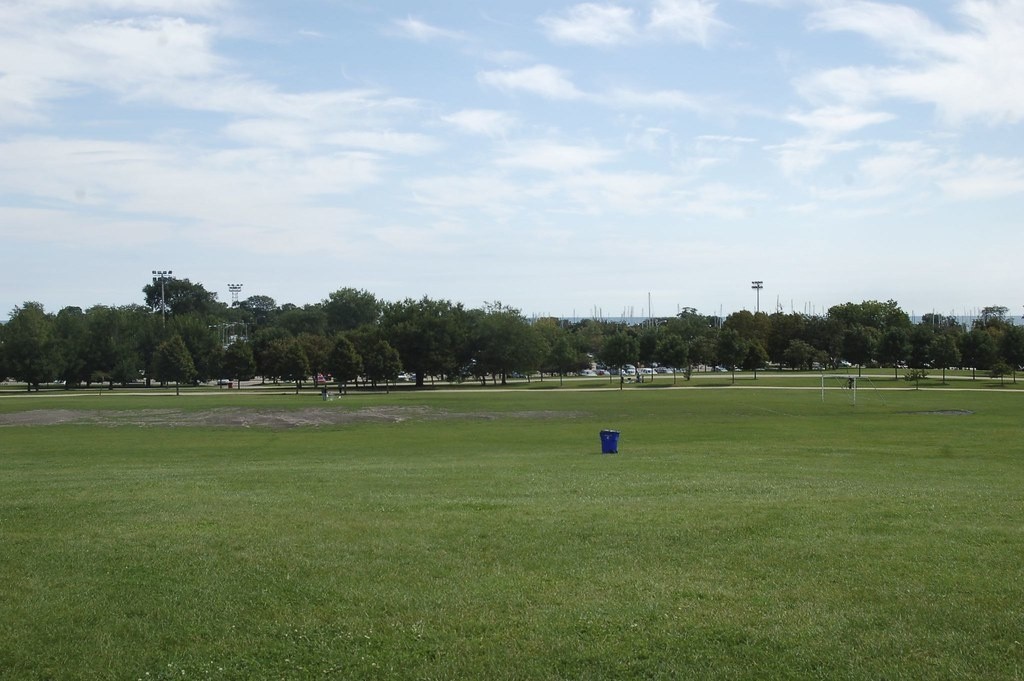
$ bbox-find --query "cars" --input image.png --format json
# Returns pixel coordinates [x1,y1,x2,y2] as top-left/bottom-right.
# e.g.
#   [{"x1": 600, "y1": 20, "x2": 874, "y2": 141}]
[
  {"x1": 581, "y1": 364, "x2": 674, "y2": 377},
  {"x1": 711, "y1": 366, "x2": 728, "y2": 372},
  {"x1": 317, "y1": 376, "x2": 326, "y2": 383}
]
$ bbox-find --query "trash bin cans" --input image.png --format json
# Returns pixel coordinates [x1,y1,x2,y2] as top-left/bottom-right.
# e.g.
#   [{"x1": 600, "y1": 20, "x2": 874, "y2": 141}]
[{"x1": 599, "y1": 429, "x2": 620, "y2": 454}]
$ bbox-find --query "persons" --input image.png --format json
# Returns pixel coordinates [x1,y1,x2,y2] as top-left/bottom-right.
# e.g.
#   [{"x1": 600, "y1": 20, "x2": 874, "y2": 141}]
[
  {"x1": 321, "y1": 384, "x2": 327, "y2": 401},
  {"x1": 847, "y1": 376, "x2": 854, "y2": 389}
]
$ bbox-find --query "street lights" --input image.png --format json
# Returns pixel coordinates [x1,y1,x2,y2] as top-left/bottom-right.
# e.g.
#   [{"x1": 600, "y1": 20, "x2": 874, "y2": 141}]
[
  {"x1": 151, "y1": 270, "x2": 172, "y2": 328},
  {"x1": 227, "y1": 282, "x2": 243, "y2": 304},
  {"x1": 751, "y1": 280, "x2": 764, "y2": 312}
]
